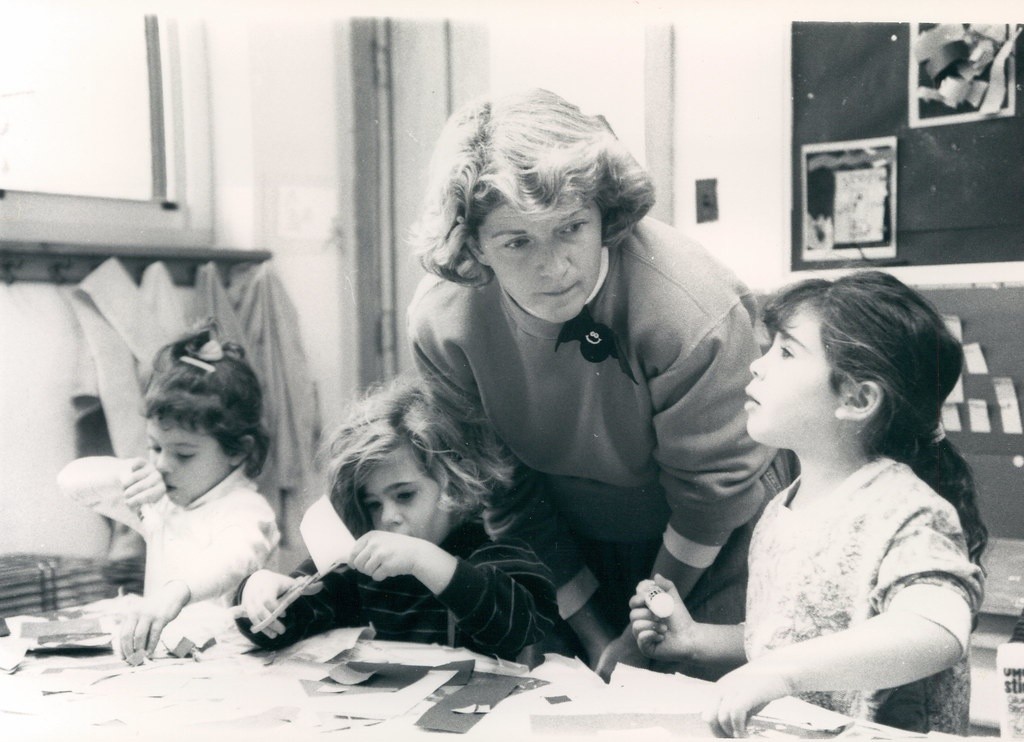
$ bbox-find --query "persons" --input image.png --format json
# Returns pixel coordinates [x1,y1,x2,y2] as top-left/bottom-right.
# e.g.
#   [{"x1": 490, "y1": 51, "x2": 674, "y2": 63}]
[
  {"x1": 628, "y1": 271, "x2": 990, "y2": 738},
  {"x1": 228, "y1": 373, "x2": 560, "y2": 664},
  {"x1": 403, "y1": 84, "x2": 799, "y2": 684},
  {"x1": 56, "y1": 331, "x2": 283, "y2": 667}
]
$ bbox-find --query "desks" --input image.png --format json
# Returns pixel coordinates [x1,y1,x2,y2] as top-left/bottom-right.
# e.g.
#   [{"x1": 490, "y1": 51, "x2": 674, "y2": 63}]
[{"x1": 0, "y1": 607, "x2": 956, "y2": 742}]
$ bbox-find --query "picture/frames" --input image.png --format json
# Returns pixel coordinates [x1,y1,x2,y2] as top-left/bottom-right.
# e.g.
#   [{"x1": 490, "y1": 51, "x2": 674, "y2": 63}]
[
  {"x1": 801, "y1": 135, "x2": 896, "y2": 262},
  {"x1": 907, "y1": 23, "x2": 1016, "y2": 130}
]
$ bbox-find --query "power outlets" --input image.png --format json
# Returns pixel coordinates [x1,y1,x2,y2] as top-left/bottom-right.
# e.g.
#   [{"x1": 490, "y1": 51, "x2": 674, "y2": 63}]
[{"x1": 694, "y1": 178, "x2": 717, "y2": 222}]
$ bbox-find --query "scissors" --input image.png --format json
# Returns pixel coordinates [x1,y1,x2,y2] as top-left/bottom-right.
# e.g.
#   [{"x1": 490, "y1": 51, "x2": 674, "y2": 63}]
[{"x1": 239, "y1": 560, "x2": 343, "y2": 635}]
